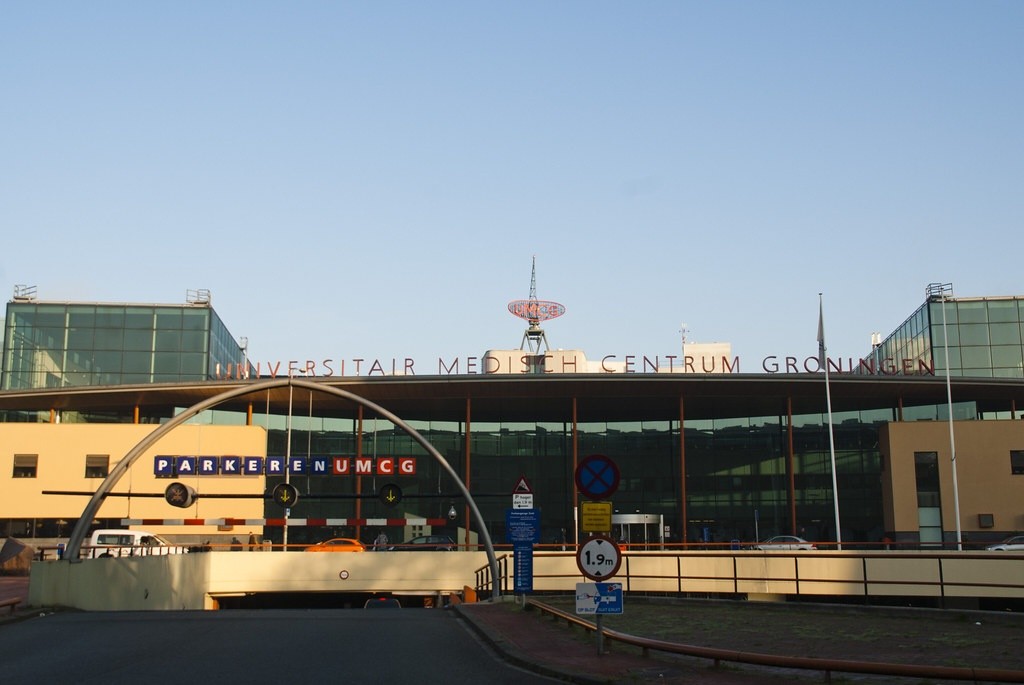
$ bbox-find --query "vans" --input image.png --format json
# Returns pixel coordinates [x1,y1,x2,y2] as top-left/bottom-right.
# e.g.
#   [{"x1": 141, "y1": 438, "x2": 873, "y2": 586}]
[{"x1": 87, "y1": 529, "x2": 189, "y2": 559}]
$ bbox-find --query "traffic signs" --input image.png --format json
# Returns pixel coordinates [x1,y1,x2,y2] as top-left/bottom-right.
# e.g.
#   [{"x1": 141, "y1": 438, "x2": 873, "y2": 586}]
[{"x1": 513, "y1": 492, "x2": 534, "y2": 509}]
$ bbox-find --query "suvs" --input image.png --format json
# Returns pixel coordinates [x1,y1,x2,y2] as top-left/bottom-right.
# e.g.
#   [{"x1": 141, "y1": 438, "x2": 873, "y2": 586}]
[
  {"x1": 387, "y1": 535, "x2": 456, "y2": 551},
  {"x1": 305, "y1": 538, "x2": 366, "y2": 552}
]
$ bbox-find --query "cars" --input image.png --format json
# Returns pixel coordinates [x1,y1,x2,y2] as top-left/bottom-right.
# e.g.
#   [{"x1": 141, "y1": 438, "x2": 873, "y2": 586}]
[
  {"x1": 985, "y1": 536, "x2": 1024, "y2": 551},
  {"x1": 363, "y1": 597, "x2": 403, "y2": 608},
  {"x1": 748, "y1": 535, "x2": 818, "y2": 550}
]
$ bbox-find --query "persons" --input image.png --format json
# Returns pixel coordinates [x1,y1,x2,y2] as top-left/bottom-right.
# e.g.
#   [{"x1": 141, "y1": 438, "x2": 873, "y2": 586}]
[
  {"x1": 230, "y1": 537, "x2": 243, "y2": 551},
  {"x1": 372, "y1": 529, "x2": 396, "y2": 550},
  {"x1": 248, "y1": 531, "x2": 256, "y2": 551},
  {"x1": 876, "y1": 534, "x2": 897, "y2": 550},
  {"x1": 205, "y1": 540, "x2": 215, "y2": 551}
]
